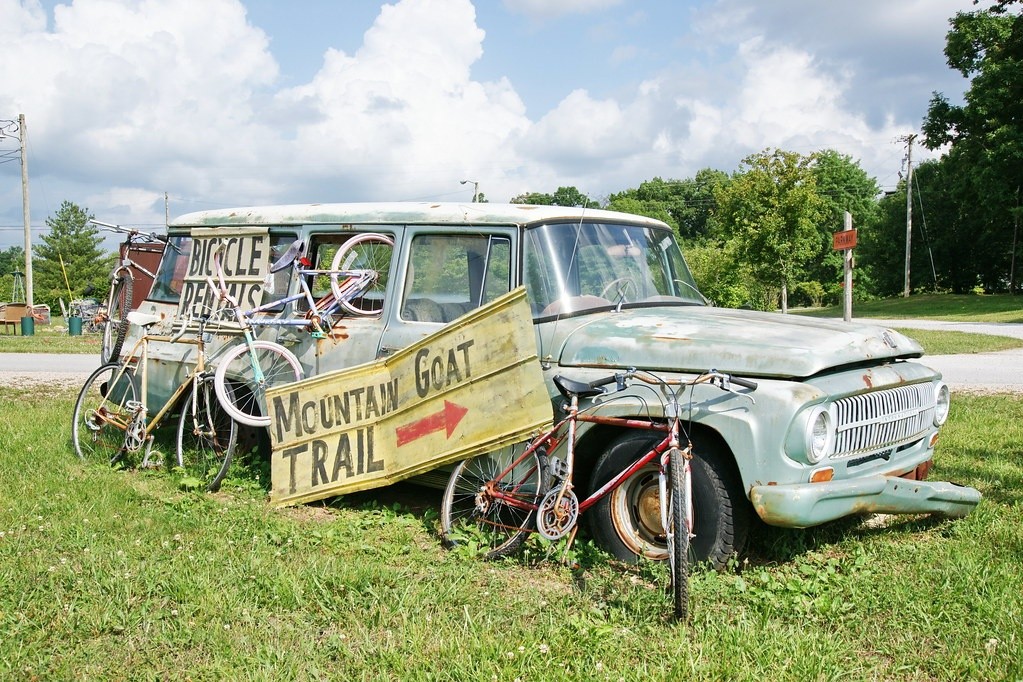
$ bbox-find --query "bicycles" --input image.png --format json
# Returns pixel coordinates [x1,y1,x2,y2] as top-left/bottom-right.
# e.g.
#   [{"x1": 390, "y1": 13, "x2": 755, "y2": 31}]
[
  {"x1": 439, "y1": 367, "x2": 759, "y2": 619},
  {"x1": 89, "y1": 219, "x2": 182, "y2": 365},
  {"x1": 71, "y1": 310, "x2": 239, "y2": 493},
  {"x1": 202, "y1": 230, "x2": 415, "y2": 426}
]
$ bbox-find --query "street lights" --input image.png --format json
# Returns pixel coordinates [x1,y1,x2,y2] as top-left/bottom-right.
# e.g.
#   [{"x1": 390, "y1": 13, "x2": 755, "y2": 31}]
[
  {"x1": 460, "y1": 179, "x2": 481, "y2": 203},
  {"x1": 0, "y1": 128, "x2": 35, "y2": 309}
]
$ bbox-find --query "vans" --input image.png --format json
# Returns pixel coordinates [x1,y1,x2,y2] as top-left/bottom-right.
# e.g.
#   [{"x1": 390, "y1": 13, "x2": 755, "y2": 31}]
[{"x1": 104, "y1": 203, "x2": 985, "y2": 574}]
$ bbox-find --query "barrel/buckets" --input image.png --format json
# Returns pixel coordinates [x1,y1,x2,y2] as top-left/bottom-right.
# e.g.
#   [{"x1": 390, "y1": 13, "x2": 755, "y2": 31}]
[
  {"x1": 20, "y1": 317, "x2": 34, "y2": 336},
  {"x1": 68, "y1": 317, "x2": 82, "y2": 336}
]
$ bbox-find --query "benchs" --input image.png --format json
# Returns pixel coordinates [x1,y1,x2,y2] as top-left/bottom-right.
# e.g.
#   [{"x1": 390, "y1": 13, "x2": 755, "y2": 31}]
[{"x1": 401, "y1": 299, "x2": 478, "y2": 322}]
